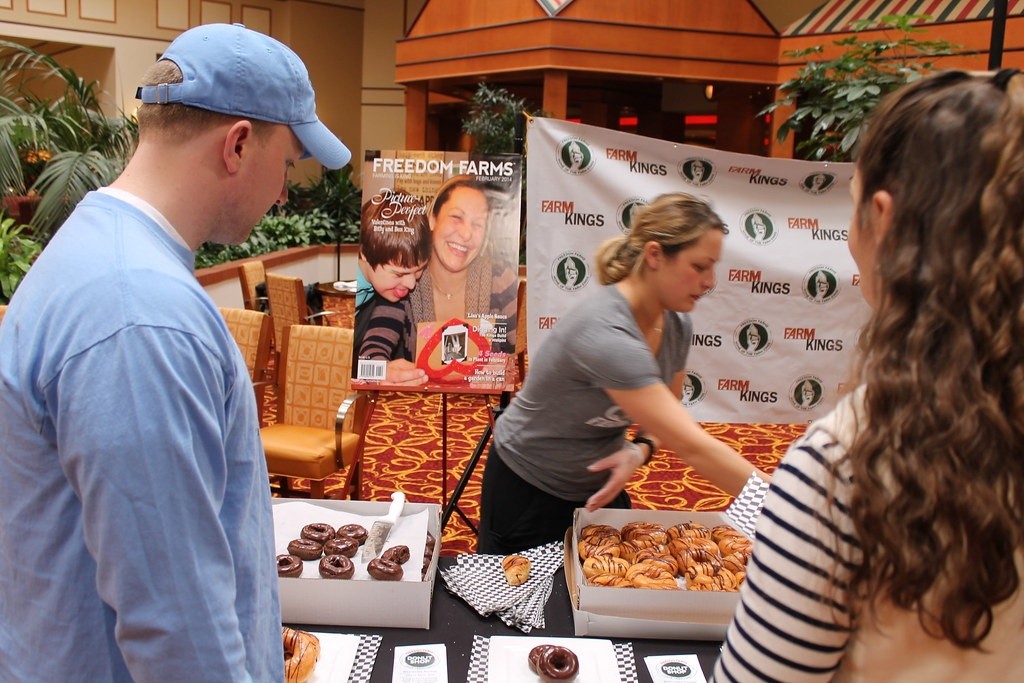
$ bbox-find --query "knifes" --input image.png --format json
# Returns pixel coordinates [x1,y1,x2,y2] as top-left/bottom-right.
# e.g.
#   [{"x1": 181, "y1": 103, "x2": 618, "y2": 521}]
[{"x1": 360, "y1": 492, "x2": 405, "y2": 563}]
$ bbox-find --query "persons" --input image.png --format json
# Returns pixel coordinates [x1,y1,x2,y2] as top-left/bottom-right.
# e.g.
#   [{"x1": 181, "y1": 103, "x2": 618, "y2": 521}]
[
  {"x1": 707, "y1": 69, "x2": 1024, "y2": 683},
  {"x1": 0, "y1": 23, "x2": 352, "y2": 682},
  {"x1": 351, "y1": 188, "x2": 432, "y2": 378},
  {"x1": 360, "y1": 174, "x2": 517, "y2": 386},
  {"x1": 478, "y1": 190, "x2": 773, "y2": 556}
]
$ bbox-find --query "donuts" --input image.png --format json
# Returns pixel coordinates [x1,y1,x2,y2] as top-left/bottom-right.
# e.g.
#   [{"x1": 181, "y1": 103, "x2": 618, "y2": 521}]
[
  {"x1": 578, "y1": 521, "x2": 753, "y2": 592},
  {"x1": 274, "y1": 522, "x2": 410, "y2": 582},
  {"x1": 281, "y1": 625, "x2": 321, "y2": 683},
  {"x1": 528, "y1": 644, "x2": 580, "y2": 683},
  {"x1": 501, "y1": 555, "x2": 531, "y2": 586}
]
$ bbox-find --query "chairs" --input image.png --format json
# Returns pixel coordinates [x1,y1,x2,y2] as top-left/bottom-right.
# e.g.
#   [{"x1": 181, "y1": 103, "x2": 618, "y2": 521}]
[
  {"x1": 264, "y1": 272, "x2": 338, "y2": 392},
  {"x1": 259, "y1": 325, "x2": 366, "y2": 503},
  {"x1": 217, "y1": 306, "x2": 271, "y2": 426},
  {"x1": 237, "y1": 259, "x2": 272, "y2": 316}
]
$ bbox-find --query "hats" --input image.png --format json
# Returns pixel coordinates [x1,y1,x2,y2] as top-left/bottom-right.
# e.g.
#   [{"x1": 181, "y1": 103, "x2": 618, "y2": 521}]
[{"x1": 134, "y1": 23, "x2": 352, "y2": 171}]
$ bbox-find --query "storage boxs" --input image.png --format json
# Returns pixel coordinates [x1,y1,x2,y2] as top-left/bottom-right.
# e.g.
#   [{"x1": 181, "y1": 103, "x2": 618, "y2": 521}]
[
  {"x1": 561, "y1": 507, "x2": 752, "y2": 643},
  {"x1": 269, "y1": 496, "x2": 442, "y2": 630}
]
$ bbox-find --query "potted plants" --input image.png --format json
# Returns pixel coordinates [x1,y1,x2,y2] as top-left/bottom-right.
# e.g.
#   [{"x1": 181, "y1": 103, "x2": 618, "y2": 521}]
[{"x1": 463, "y1": 80, "x2": 542, "y2": 191}]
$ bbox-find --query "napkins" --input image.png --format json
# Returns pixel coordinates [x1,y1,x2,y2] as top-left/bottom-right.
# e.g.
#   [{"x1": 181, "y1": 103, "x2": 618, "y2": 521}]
[
  {"x1": 390, "y1": 643, "x2": 448, "y2": 683},
  {"x1": 644, "y1": 653, "x2": 708, "y2": 683}
]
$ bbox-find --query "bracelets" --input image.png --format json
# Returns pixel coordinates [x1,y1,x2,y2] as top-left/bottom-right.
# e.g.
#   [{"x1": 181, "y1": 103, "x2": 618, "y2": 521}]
[{"x1": 632, "y1": 436, "x2": 655, "y2": 467}]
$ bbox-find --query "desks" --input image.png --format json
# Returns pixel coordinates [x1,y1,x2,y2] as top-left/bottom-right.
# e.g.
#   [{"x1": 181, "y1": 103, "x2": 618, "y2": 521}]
[
  {"x1": 315, "y1": 280, "x2": 357, "y2": 331},
  {"x1": 336, "y1": 377, "x2": 507, "y2": 503},
  {"x1": 275, "y1": 552, "x2": 724, "y2": 683}
]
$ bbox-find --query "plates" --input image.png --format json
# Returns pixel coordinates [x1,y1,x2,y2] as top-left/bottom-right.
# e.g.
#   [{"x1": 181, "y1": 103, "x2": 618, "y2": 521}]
[
  {"x1": 488, "y1": 635, "x2": 622, "y2": 683},
  {"x1": 305, "y1": 631, "x2": 361, "y2": 683}
]
